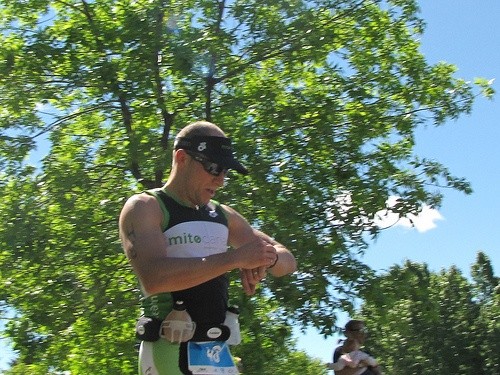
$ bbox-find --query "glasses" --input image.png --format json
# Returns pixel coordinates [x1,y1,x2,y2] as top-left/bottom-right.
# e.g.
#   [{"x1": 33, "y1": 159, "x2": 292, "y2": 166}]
[
  {"x1": 187, "y1": 153, "x2": 229, "y2": 176},
  {"x1": 349, "y1": 328, "x2": 368, "y2": 333}
]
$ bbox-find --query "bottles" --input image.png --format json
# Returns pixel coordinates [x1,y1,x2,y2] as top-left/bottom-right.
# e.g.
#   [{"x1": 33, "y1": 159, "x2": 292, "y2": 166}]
[
  {"x1": 226, "y1": 305, "x2": 240, "y2": 347},
  {"x1": 163, "y1": 300, "x2": 193, "y2": 342}
]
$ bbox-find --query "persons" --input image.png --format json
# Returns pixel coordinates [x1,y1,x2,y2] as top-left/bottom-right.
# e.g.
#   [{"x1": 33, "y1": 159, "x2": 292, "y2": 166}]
[
  {"x1": 325, "y1": 338, "x2": 378, "y2": 375},
  {"x1": 333, "y1": 320, "x2": 381, "y2": 375},
  {"x1": 119, "y1": 120, "x2": 296, "y2": 375}
]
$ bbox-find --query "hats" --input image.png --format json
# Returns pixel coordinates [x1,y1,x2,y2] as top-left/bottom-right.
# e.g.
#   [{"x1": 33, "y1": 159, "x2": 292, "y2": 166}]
[{"x1": 174, "y1": 135, "x2": 249, "y2": 178}]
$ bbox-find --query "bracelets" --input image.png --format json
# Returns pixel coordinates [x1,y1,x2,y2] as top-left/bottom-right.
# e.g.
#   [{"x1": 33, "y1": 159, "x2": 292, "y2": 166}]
[{"x1": 267, "y1": 252, "x2": 279, "y2": 269}]
